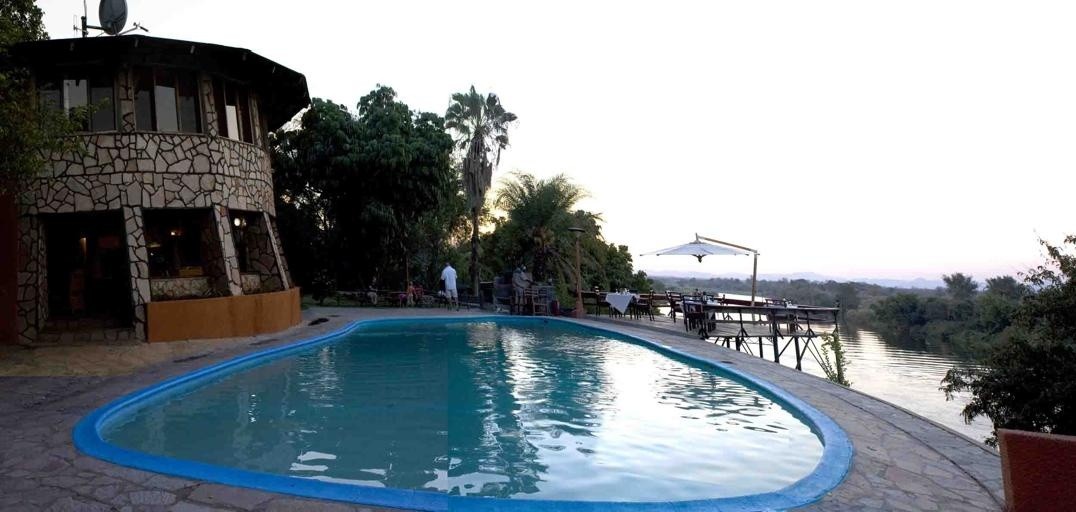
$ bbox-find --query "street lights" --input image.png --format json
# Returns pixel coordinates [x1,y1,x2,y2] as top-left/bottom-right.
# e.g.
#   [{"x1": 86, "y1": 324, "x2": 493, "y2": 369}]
[{"x1": 566, "y1": 227, "x2": 588, "y2": 318}]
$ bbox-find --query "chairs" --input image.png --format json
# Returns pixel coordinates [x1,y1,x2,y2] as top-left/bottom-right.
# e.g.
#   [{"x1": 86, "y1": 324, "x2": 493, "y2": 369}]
[{"x1": 493, "y1": 285, "x2": 802, "y2": 337}]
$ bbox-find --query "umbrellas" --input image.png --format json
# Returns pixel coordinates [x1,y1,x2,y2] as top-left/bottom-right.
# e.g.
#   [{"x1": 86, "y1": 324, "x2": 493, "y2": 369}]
[{"x1": 640, "y1": 233, "x2": 761, "y2": 306}]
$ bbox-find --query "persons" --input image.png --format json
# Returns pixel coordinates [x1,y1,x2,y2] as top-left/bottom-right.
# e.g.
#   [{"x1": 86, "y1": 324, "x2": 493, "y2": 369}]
[
  {"x1": 366, "y1": 276, "x2": 379, "y2": 307},
  {"x1": 700, "y1": 292, "x2": 709, "y2": 302},
  {"x1": 693, "y1": 289, "x2": 701, "y2": 300},
  {"x1": 512, "y1": 264, "x2": 538, "y2": 315},
  {"x1": 399, "y1": 282, "x2": 424, "y2": 308},
  {"x1": 441, "y1": 262, "x2": 460, "y2": 311}
]
